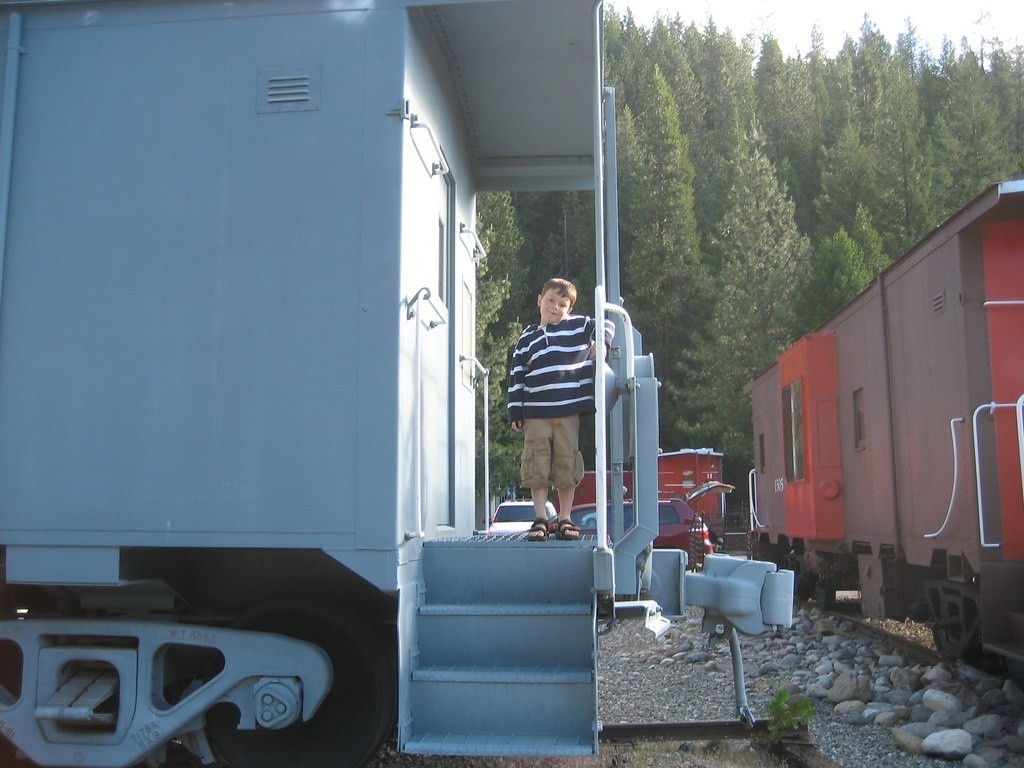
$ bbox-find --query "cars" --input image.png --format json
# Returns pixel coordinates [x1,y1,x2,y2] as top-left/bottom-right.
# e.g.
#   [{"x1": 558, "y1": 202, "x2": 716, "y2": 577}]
[
  {"x1": 547, "y1": 480, "x2": 736, "y2": 570},
  {"x1": 488, "y1": 497, "x2": 558, "y2": 535}
]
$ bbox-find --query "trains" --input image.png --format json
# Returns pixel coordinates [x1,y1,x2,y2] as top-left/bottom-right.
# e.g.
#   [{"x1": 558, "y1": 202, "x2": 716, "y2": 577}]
[
  {"x1": 0, "y1": 0, "x2": 795, "y2": 768},
  {"x1": 741, "y1": 180, "x2": 1023, "y2": 666},
  {"x1": 557, "y1": 447, "x2": 727, "y2": 552}
]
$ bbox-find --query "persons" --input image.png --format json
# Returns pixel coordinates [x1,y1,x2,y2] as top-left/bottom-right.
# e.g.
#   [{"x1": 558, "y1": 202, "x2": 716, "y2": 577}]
[{"x1": 508, "y1": 278, "x2": 616, "y2": 541}]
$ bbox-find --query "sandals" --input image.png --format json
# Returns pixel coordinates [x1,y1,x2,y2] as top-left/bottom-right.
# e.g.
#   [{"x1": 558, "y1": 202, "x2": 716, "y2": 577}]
[
  {"x1": 529, "y1": 518, "x2": 549, "y2": 540},
  {"x1": 556, "y1": 520, "x2": 580, "y2": 540}
]
